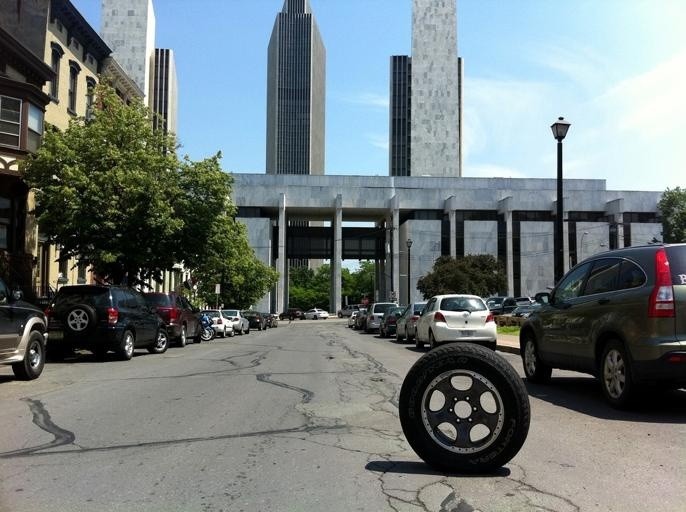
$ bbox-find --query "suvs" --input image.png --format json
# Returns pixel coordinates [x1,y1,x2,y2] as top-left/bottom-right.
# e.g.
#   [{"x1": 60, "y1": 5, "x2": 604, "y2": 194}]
[
  {"x1": 0, "y1": 276, "x2": 49, "y2": 379},
  {"x1": 337, "y1": 304, "x2": 368, "y2": 319},
  {"x1": 279, "y1": 307, "x2": 304, "y2": 321},
  {"x1": 520, "y1": 243, "x2": 685, "y2": 403}
]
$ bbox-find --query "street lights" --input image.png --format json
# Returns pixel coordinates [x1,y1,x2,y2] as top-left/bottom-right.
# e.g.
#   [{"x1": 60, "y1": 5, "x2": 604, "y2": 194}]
[
  {"x1": 406, "y1": 238, "x2": 412, "y2": 305},
  {"x1": 551, "y1": 115, "x2": 571, "y2": 285}
]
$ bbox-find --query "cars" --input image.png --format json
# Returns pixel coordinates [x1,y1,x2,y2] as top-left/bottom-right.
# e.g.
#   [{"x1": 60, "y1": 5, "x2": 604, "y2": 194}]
[
  {"x1": 349, "y1": 292, "x2": 498, "y2": 351},
  {"x1": 483, "y1": 295, "x2": 543, "y2": 326},
  {"x1": 45, "y1": 285, "x2": 278, "y2": 360},
  {"x1": 304, "y1": 308, "x2": 330, "y2": 320}
]
